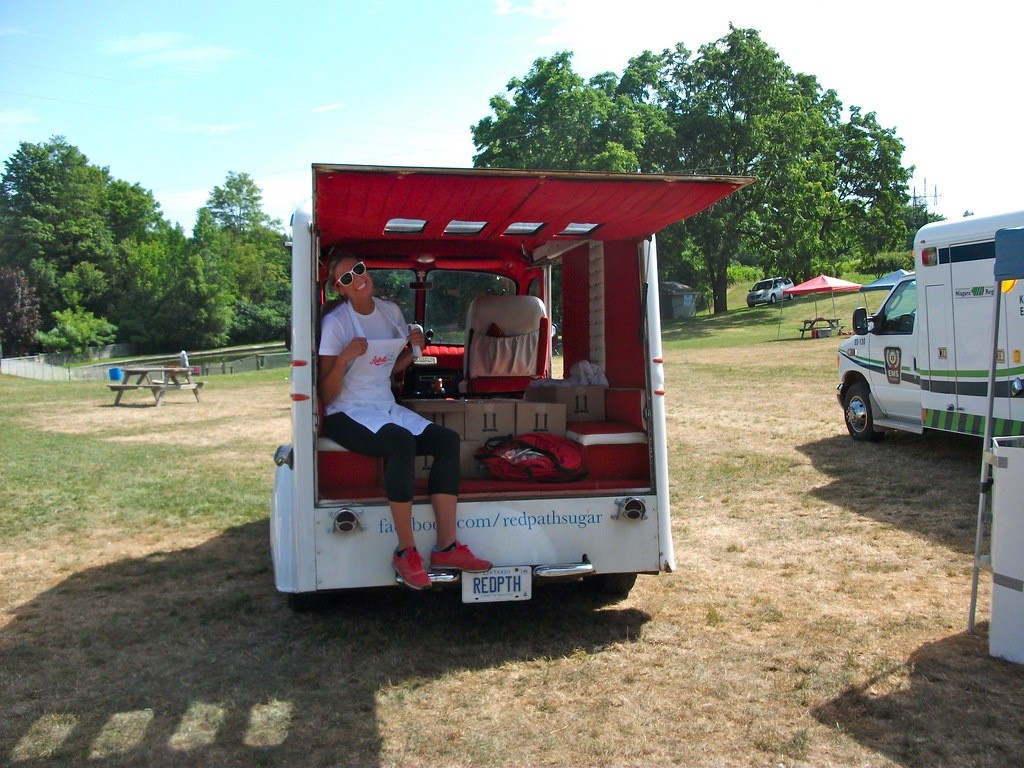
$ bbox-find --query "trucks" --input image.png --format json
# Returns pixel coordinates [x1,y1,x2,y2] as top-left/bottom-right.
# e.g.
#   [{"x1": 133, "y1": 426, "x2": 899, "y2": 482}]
[
  {"x1": 269, "y1": 159, "x2": 758, "y2": 610},
  {"x1": 832, "y1": 210, "x2": 1024, "y2": 444}
]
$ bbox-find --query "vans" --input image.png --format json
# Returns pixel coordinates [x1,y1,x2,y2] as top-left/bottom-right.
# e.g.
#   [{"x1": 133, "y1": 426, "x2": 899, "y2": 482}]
[{"x1": 747, "y1": 276, "x2": 795, "y2": 307}]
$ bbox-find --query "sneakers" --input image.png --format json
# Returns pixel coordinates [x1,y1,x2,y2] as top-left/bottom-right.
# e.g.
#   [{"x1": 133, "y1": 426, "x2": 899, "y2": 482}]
[
  {"x1": 430, "y1": 542, "x2": 492, "y2": 573},
  {"x1": 391, "y1": 545, "x2": 433, "y2": 590}
]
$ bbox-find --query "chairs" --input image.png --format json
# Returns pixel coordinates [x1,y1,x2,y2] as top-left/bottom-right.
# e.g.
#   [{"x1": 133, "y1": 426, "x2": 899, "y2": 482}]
[
  {"x1": 565, "y1": 386, "x2": 646, "y2": 480},
  {"x1": 460, "y1": 297, "x2": 553, "y2": 399},
  {"x1": 315, "y1": 397, "x2": 388, "y2": 499}
]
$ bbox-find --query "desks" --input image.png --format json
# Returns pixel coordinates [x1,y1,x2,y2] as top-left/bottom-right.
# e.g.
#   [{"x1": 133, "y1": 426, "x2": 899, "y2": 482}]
[
  {"x1": 800, "y1": 318, "x2": 841, "y2": 338},
  {"x1": 115, "y1": 365, "x2": 201, "y2": 408}
]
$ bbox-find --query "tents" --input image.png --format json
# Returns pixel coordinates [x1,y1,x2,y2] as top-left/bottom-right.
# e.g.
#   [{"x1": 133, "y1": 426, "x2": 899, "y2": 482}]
[
  {"x1": 857, "y1": 269, "x2": 916, "y2": 307},
  {"x1": 777, "y1": 276, "x2": 869, "y2": 339}
]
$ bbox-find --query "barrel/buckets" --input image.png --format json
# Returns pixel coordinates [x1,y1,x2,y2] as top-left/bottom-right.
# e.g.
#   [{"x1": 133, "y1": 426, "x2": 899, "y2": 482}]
[
  {"x1": 109, "y1": 367, "x2": 123, "y2": 380},
  {"x1": 819, "y1": 328, "x2": 832, "y2": 337}
]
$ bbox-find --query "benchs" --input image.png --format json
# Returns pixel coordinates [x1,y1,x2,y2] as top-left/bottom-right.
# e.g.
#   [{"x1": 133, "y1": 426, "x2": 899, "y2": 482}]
[
  {"x1": 107, "y1": 380, "x2": 205, "y2": 407},
  {"x1": 798, "y1": 325, "x2": 847, "y2": 339}
]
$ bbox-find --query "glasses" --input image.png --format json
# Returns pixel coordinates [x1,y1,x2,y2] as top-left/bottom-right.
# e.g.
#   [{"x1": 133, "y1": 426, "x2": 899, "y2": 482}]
[{"x1": 334, "y1": 261, "x2": 366, "y2": 286}]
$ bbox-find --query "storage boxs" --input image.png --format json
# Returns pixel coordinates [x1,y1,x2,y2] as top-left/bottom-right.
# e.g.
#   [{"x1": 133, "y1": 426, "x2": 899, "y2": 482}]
[{"x1": 401, "y1": 382, "x2": 605, "y2": 481}]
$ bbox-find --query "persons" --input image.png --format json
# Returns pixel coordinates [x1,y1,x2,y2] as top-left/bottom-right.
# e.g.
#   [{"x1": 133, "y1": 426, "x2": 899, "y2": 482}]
[
  {"x1": 968, "y1": 226, "x2": 1024, "y2": 634},
  {"x1": 856, "y1": 316, "x2": 865, "y2": 328},
  {"x1": 318, "y1": 252, "x2": 492, "y2": 590}
]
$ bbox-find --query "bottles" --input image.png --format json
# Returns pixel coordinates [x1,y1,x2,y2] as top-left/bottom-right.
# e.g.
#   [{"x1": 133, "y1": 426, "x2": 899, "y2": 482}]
[{"x1": 181, "y1": 350, "x2": 189, "y2": 367}]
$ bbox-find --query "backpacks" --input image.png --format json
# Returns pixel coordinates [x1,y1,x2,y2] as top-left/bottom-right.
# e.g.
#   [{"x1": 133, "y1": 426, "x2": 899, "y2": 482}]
[{"x1": 473, "y1": 428, "x2": 589, "y2": 481}]
